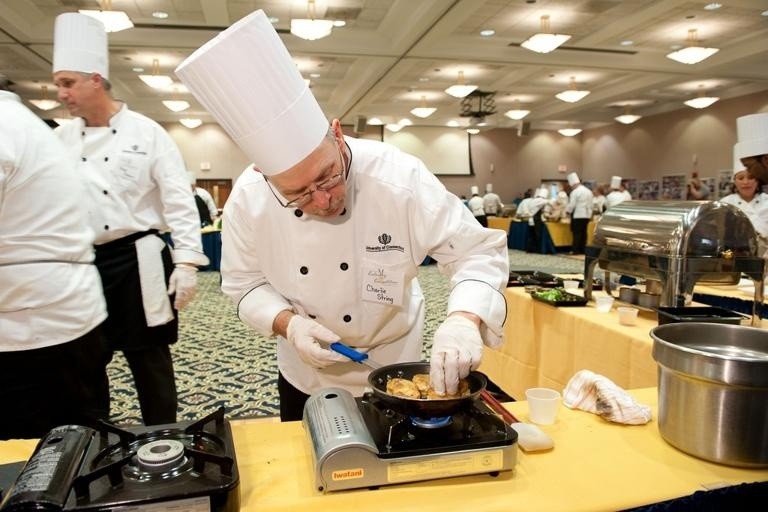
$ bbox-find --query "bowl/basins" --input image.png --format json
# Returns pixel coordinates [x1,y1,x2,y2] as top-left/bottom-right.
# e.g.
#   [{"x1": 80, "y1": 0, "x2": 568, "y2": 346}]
[{"x1": 649, "y1": 321, "x2": 768, "y2": 473}]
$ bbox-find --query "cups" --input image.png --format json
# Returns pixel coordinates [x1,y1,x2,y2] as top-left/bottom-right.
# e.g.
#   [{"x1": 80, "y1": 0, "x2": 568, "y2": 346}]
[
  {"x1": 594, "y1": 294, "x2": 614, "y2": 313},
  {"x1": 525, "y1": 387, "x2": 561, "y2": 425},
  {"x1": 617, "y1": 286, "x2": 639, "y2": 304},
  {"x1": 638, "y1": 292, "x2": 660, "y2": 310},
  {"x1": 617, "y1": 305, "x2": 636, "y2": 325},
  {"x1": 563, "y1": 279, "x2": 579, "y2": 293}
]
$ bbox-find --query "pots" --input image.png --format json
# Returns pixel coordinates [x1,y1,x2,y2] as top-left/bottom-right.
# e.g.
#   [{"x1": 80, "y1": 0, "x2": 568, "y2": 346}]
[{"x1": 326, "y1": 337, "x2": 487, "y2": 416}]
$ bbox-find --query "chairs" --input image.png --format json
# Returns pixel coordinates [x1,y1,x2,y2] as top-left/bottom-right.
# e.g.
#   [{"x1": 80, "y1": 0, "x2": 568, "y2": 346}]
[{"x1": 195, "y1": 218, "x2": 222, "y2": 270}]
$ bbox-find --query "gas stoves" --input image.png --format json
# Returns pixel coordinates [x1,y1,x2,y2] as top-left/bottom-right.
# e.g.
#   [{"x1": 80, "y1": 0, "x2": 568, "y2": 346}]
[
  {"x1": 302, "y1": 387, "x2": 519, "y2": 495},
  {"x1": 1, "y1": 408, "x2": 241, "y2": 511}
]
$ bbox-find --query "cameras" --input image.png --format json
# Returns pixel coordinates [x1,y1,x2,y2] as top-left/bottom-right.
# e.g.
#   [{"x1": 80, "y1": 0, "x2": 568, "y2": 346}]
[{"x1": 688, "y1": 183, "x2": 691, "y2": 188}]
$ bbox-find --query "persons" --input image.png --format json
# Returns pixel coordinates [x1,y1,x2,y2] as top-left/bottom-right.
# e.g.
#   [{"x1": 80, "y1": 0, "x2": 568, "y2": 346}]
[
  {"x1": 191, "y1": 184, "x2": 219, "y2": 228},
  {"x1": 461, "y1": 183, "x2": 633, "y2": 255},
  {"x1": 687, "y1": 178, "x2": 709, "y2": 200},
  {"x1": 0, "y1": 91, "x2": 113, "y2": 441},
  {"x1": 719, "y1": 170, "x2": 768, "y2": 280},
  {"x1": 739, "y1": 154, "x2": 768, "y2": 184},
  {"x1": 52, "y1": 71, "x2": 212, "y2": 426},
  {"x1": 220, "y1": 119, "x2": 510, "y2": 422}
]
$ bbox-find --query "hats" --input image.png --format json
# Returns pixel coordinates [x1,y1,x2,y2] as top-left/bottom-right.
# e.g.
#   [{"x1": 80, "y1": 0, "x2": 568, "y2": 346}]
[
  {"x1": 736, "y1": 113, "x2": 768, "y2": 159},
  {"x1": 52, "y1": 12, "x2": 109, "y2": 81},
  {"x1": 174, "y1": 8, "x2": 330, "y2": 176},
  {"x1": 471, "y1": 183, "x2": 567, "y2": 204},
  {"x1": 732, "y1": 142, "x2": 747, "y2": 180},
  {"x1": 566, "y1": 172, "x2": 580, "y2": 186},
  {"x1": 610, "y1": 176, "x2": 622, "y2": 190},
  {"x1": 187, "y1": 170, "x2": 197, "y2": 185}
]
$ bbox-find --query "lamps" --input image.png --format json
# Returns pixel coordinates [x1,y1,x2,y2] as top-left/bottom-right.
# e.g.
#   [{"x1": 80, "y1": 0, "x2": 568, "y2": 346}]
[
  {"x1": 466, "y1": 127, "x2": 482, "y2": 136},
  {"x1": 161, "y1": 87, "x2": 190, "y2": 112},
  {"x1": 177, "y1": 109, "x2": 204, "y2": 130},
  {"x1": 409, "y1": 96, "x2": 438, "y2": 118},
  {"x1": 446, "y1": 119, "x2": 457, "y2": 127},
  {"x1": 139, "y1": 59, "x2": 176, "y2": 93},
  {"x1": 521, "y1": 14, "x2": 570, "y2": 55},
  {"x1": 666, "y1": 27, "x2": 719, "y2": 66},
  {"x1": 365, "y1": 115, "x2": 383, "y2": 126},
  {"x1": 444, "y1": 71, "x2": 477, "y2": 97},
  {"x1": 385, "y1": 120, "x2": 404, "y2": 133},
  {"x1": 554, "y1": 76, "x2": 590, "y2": 103},
  {"x1": 556, "y1": 121, "x2": 582, "y2": 137},
  {"x1": 289, "y1": 3, "x2": 335, "y2": 43},
  {"x1": 33, "y1": 87, "x2": 61, "y2": 113},
  {"x1": 614, "y1": 105, "x2": 643, "y2": 125},
  {"x1": 397, "y1": 116, "x2": 413, "y2": 126},
  {"x1": 503, "y1": 98, "x2": 531, "y2": 122},
  {"x1": 78, "y1": 7, "x2": 133, "y2": 41},
  {"x1": 477, "y1": 122, "x2": 487, "y2": 127},
  {"x1": 682, "y1": 82, "x2": 720, "y2": 110}
]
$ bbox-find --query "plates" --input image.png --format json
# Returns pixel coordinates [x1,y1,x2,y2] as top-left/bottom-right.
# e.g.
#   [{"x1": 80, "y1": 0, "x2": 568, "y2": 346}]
[
  {"x1": 530, "y1": 289, "x2": 587, "y2": 307},
  {"x1": 505, "y1": 268, "x2": 561, "y2": 288}
]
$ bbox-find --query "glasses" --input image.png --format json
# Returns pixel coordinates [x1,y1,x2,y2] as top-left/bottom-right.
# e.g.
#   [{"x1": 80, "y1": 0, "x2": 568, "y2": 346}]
[{"x1": 263, "y1": 138, "x2": 344, "y2": 208}]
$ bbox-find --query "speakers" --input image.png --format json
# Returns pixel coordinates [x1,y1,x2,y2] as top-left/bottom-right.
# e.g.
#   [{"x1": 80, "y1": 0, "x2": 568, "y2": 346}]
[
  {"x1": 354, "y1": 115, "x2": 367, "y2": 133},
  {"x1": 517, "y1": 119, "x2": 530, "y2": 136}
]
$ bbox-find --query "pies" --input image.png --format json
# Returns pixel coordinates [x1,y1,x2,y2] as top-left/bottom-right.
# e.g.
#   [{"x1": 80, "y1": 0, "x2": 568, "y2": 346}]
[{"x1": 387, "y1": 374, "x2": 470, "y2": 400}]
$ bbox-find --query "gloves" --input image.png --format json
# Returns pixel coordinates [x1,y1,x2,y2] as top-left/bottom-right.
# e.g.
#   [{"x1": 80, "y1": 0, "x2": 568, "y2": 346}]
[
  {"x1": 167, "y1": 264, "x2": 199, "y2": 312},
  {"x1": 286, "y1": 315, "x2": 352, "y2": 369},
  {"x1": 429, "y1": 315, "x2": 484, "y2": 398}
]
